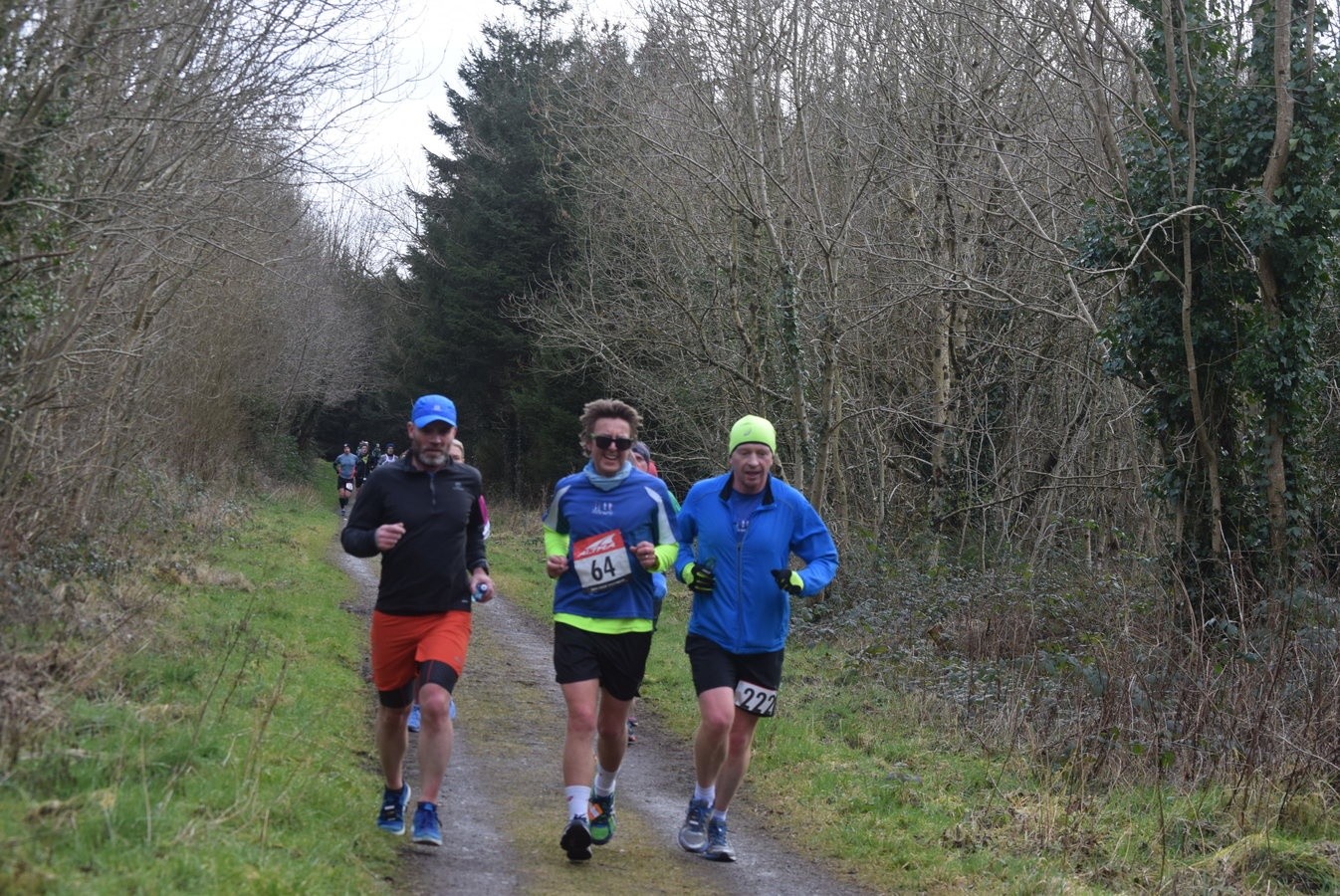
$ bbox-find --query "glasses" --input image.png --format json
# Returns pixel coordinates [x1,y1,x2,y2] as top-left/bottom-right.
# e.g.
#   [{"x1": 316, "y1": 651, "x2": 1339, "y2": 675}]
[{"x1": 585, "y1": 433, "x2": 634, "y2": 451}]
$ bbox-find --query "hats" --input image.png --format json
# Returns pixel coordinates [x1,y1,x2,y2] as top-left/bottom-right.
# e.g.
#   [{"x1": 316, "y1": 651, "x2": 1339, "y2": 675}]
[
  {"x1": 648, "y1": 459, "x2": 658, "y2": 477},
  {"x1": 386, "y1": 442, "x2": 394, "y2": 449},
  {"x1": 730, "y1": 414, "x2": 776, "y2": 456},
  {"x1": 632, "y1": 441, "x2": 651, "y2": 464},
  {"x1": 344, "y1": 443, "x2": 351, "y2": 448},
  {"x1": 411, "y1": 395, "x2": 457, "y2": 428}
]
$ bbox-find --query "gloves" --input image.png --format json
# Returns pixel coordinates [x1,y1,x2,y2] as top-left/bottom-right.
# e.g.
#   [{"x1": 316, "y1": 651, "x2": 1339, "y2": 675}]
[
  {"x1": 771, "y1": 569, "x2": 804, "y2": 594},
  {"x1": 681, "y1": 561, "x2": 715, "y2": 595}
]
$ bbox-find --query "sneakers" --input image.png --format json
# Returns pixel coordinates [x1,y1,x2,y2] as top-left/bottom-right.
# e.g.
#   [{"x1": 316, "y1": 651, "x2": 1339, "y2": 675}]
[
  {"x1": 377, "y1": 779, "x2": 411, "y2": 836},
  {"x1": 700, "y1": 816, "x2": 736, "y2": 861},
  {"x1": 625, "y1": 717, "x2": 639, "y2": 744},
  {"x1": 450, "y1": 700, "x2": 455, "y2": 719},
  {"x1": 588, "y1": 785, "x2": 615, "y2": 846},
  {"x1": 412, "y1": 807, "x2": 444, "y2": 847},
  {"x1": 559, "y1": 813, "x2": 592, "y2": 861},
  {"x1": 407, "y1": 705, "x2": 421, "y2": 732},
  {"x1": 679, "y1": 792, "x2": 716, "y2": 853}
]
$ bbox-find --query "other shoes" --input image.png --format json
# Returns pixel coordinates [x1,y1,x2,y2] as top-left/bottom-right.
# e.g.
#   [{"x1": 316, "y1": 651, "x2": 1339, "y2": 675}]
[{"x1": 341, "y1": 510, "x2": 345, "y2": 516}]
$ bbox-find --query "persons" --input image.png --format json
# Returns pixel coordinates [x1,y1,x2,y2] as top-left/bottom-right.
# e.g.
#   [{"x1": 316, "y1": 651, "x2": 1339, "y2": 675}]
[
  {"x1": 673, "y1": 414, "x2": 840, "y2": 865},
  {"x1": 330, "y1": 437, "x2": 415, "y2": 526},
  {"x1": 541, "y1": 398, "x2": 681, "y2": 863},
  {"x1": 338, "y1": 393, "x2": 497, "y2": 847},
  {"x1": 610, "y1": 437, "x2": 674, "y2": 746},
  {"x1": 407, "y1": 439, "x2": 491, "y2": 736}
]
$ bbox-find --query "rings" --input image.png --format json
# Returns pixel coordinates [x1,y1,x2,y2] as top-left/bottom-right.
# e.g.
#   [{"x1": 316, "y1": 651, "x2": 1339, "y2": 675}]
[{"x1": 644, "y1": 553, "x2": 648, "y2": 559}]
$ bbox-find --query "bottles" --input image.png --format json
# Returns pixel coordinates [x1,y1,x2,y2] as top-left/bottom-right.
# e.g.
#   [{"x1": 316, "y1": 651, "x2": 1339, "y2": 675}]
[{"x1": 472, "y1": 583, "x2": 489, "y2": 602}]
